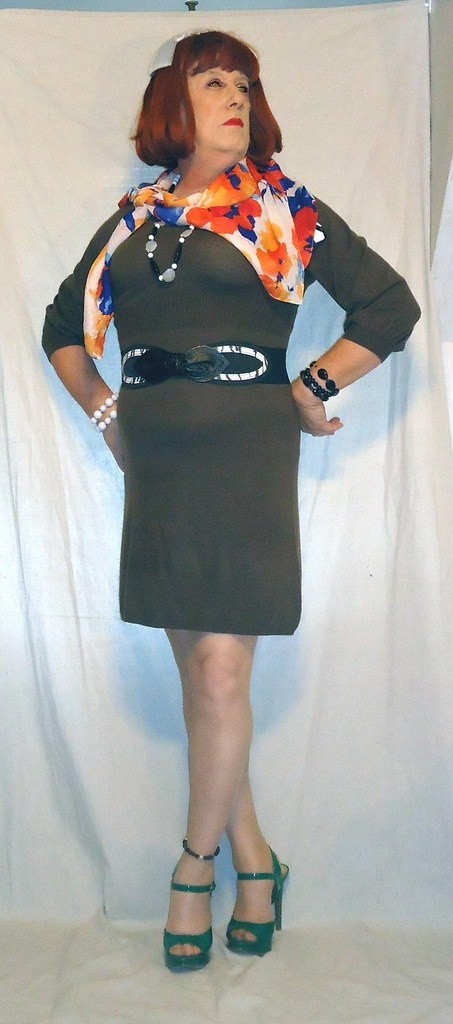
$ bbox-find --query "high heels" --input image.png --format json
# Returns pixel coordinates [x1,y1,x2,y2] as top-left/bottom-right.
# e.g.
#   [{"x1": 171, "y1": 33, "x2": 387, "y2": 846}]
[
  {"x1": 226, "y1": 845, "x2": 289, "y2": 954},
  {"x1": 163, "y1": 866, "x2": 216, "y2": 972}
]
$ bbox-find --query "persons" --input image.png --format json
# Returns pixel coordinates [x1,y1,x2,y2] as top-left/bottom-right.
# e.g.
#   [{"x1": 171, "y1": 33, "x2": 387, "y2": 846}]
[{"x1": 41, "y1": 29, "x2": 421, "y2": 974}]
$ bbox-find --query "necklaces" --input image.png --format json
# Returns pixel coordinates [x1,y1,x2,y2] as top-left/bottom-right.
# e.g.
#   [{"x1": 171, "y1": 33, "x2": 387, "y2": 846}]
[{"x1": 144, "y1": 173, "x2": 196, "y2": 283}]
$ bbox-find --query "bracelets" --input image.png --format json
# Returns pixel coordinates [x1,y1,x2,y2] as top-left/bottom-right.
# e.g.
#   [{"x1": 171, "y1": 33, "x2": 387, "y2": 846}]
[
  {"x1": 300, "y1": 360, "x2": 339, "y2": 402},
  {"x1": 89, "y1": 392, "x2": 120, "y2": 424},
  {"x1": 94, "y1": 410, "x2": 117, "y2": 434}
]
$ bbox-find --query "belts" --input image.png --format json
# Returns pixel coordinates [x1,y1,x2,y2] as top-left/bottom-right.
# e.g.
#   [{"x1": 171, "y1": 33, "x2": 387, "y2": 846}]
[{"x1": 120, "y1": 342, "x2": 290, "y2": 390}]
